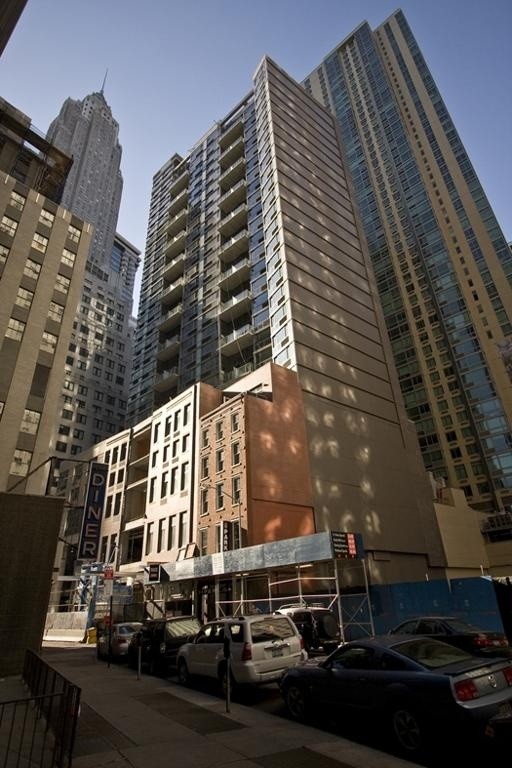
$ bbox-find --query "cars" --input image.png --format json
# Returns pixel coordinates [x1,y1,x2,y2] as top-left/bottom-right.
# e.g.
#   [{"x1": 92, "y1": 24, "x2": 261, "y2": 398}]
[
  {"x1": 279, "y1": 617, "x2": 512, "y2": 752},
  {"x1": 98, "y1": 622, "x2": 144, "y2": 656}
]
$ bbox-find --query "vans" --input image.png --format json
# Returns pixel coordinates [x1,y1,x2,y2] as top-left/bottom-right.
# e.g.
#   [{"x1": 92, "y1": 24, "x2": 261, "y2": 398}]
[{"x1": 127, "y1": 615, "x2": 203, "y2": 672}]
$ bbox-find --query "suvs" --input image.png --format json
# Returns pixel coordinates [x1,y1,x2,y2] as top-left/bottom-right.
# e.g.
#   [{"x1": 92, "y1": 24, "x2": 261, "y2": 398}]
[
  {"x1": 273, "y1": 603, "x2": 341, "y2": 655},
  {"x1": 176, "y1": 615, "x2": 309, "y2": 697}
]
